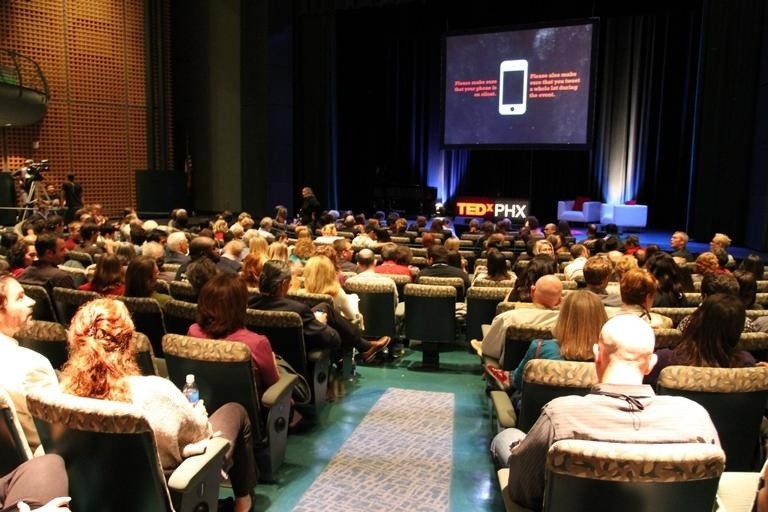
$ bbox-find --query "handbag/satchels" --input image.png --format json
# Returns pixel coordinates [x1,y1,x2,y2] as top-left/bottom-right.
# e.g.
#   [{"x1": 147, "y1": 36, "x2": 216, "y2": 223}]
[{"x1": 275, "y1": 355, "x2": 312, "y2": 406}]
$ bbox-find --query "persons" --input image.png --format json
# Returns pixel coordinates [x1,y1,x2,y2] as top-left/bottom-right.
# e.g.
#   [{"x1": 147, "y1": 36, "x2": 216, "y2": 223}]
[
  {"x1": 39, "y1": 183, "x2": 61, "y2": 205},
  {"x1": 301, "y1": 187, "x2": 321, "y2": 223},
  {"x1": 0, "y1": 453, "x2": 72, "y2": 512},
  {"x1": 12, "y1": 161, "x2": 44, "y2": 206},
  {"x1": 63, "y1": 173, "x2": 82, "y2": 219},
  {"x1": 491, "y1": 314, "x2": 722, "y2": 512},
  {"x1": 54, "y1": 298, "x2": 259, "y2": 512},
  {"x1": 0, "y1": 207, "x2": 768, "y2": 435}
]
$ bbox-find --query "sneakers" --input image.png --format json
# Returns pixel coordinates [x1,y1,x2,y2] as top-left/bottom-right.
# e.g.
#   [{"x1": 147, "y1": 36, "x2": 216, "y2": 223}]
[
  {"x1": 486, "y1": 362, "x2": 511, "y2": 391},
  {"x1": 470, "y1": 339, "x2": 483, "y2": 359}
]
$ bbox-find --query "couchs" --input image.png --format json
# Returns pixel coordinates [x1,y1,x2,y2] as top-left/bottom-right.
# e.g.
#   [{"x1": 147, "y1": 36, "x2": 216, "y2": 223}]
[
  {"x1": 600, "y1": 204, "x2": 648, "y2": 232},
  {"x1": 557, "y1": 200, "x2": 601, "y2": 233}
]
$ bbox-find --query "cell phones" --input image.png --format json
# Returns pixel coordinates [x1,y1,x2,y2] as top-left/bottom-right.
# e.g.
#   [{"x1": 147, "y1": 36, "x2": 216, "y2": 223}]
[{"x1": 499, "y1": 59, "x2": 528, "y2": 116}]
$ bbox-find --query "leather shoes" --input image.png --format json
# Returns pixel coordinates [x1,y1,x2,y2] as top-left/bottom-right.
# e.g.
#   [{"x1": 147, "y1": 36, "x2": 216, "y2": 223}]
[
  {"x1": 231, "y1": 488, "x2": 257, "y2": 511},
  {"x1": 361, "y1": 336, "x2": 392, "y2": 364},
  {"x1": 195, "y1": 496, "x2": 232, "y2": 511}
]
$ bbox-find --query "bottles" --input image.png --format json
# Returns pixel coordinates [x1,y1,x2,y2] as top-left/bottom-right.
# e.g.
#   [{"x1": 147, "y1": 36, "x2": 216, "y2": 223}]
[{"x1": 182, "y1": 374, "x2": 199, "y2": 403}]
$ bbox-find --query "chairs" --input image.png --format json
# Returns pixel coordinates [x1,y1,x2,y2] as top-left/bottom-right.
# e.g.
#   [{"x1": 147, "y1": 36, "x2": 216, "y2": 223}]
[
  {"x1": 495, "y1": 439, "x2": 727, "y2": 510},
  {"x1": 0, "y1": 392, "x2": 46, "y2": 475},
  {"x1": 490, "y1": 359, "x2": 598, "y2": 444},
  {"x1": 716, "y1": 456, "x2": 768, "y2": 511},
  {"x1": 162, "y1": 332, "x2": 297, "y2": 482},
  {"x1": 24, "y1": 395, "x2": 234, "y2": 511},
  {"x1": 655, "y1": 365, "x2": 768, "y2": 470}
]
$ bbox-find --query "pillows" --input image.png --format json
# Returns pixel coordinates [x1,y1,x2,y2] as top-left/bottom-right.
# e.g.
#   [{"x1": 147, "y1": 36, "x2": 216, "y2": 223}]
[
  {"x1": 572, "y1": 197, "x2": 591, "y2": 211},
  {"x1": 624, "y1": 200, "x2": 636, "y2": 205}
]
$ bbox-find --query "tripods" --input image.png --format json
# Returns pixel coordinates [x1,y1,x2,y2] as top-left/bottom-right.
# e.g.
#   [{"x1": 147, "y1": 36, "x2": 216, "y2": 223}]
[{"x1": 22, "y1": 182, "x2": 58, "y2": 219}]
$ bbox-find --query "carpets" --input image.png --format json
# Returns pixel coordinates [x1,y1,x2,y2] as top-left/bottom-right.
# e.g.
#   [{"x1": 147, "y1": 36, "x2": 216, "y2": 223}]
[{"x1": 292, "y1": 386, "x2": 456, "y2": 510}]
